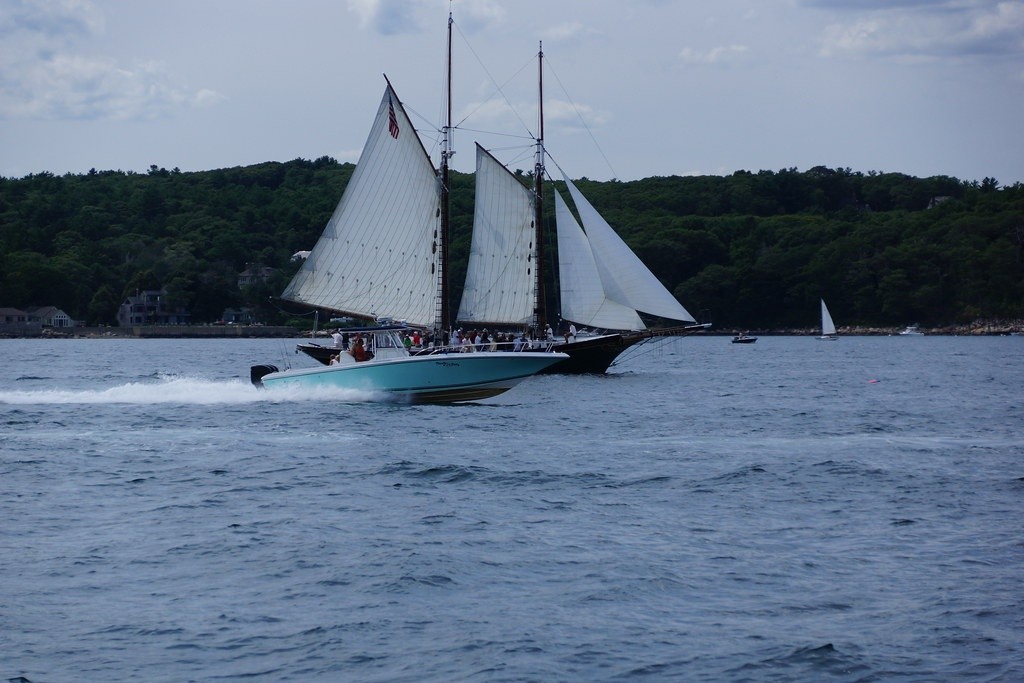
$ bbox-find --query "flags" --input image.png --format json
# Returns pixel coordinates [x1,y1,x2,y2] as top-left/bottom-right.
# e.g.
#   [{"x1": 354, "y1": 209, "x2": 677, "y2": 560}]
[{"x1": 389, "y1": 93, "x2": 399, "y2": 139}]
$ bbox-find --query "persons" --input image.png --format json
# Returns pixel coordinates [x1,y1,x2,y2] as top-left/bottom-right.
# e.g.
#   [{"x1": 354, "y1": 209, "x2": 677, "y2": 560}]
[
  {"x1": 398, "y1": 327, "x2": 529, "y2": 353},
  {"x1": 564, "y1": 321, "x2": 577, "y2": 344},
  {"x1": 544, "y1": 324, "x2": 554, "y2": 346},
  {"x1": 739, "y1": 332, "x2": 742, "y2": 339},
  {"x1": 328, "y1": 329, "x2": 378, "y2": 367}
]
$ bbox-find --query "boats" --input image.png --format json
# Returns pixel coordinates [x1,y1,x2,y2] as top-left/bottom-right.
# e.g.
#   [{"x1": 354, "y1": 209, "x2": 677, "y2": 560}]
[
  {"x1": 731, "y1": 337, "x2": 759, "y2": 344},
  {"x1": 1011, "y1": 333, "x2": 1022, "y2": 336},
  {"x1": 251, "y1": 322, "x2": 571, "y2": 407},
  {"x1": 900, "y1": 326, "x2": 924, "y2": 337}
]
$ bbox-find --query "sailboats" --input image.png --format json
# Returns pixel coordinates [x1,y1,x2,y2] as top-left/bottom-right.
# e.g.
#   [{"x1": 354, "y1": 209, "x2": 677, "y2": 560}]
[
  {"x1": 814, "y1": 299, "x2": 840, "y2": 341},
  {"x1": 300, "y1": 38, "x2": 712, "y2": 377}
]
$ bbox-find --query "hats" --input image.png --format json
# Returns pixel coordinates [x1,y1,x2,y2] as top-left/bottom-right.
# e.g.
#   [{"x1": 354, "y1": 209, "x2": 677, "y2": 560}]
[
  {"x1": 477, "y1": 332, "x2": 481, "y2": 334},
  {"x1": 483, "y1": 328, "x2": 488, "y2": 332},
  {"x1": 406, "y1": 334, "x2": 409, "y2": 336}
]
